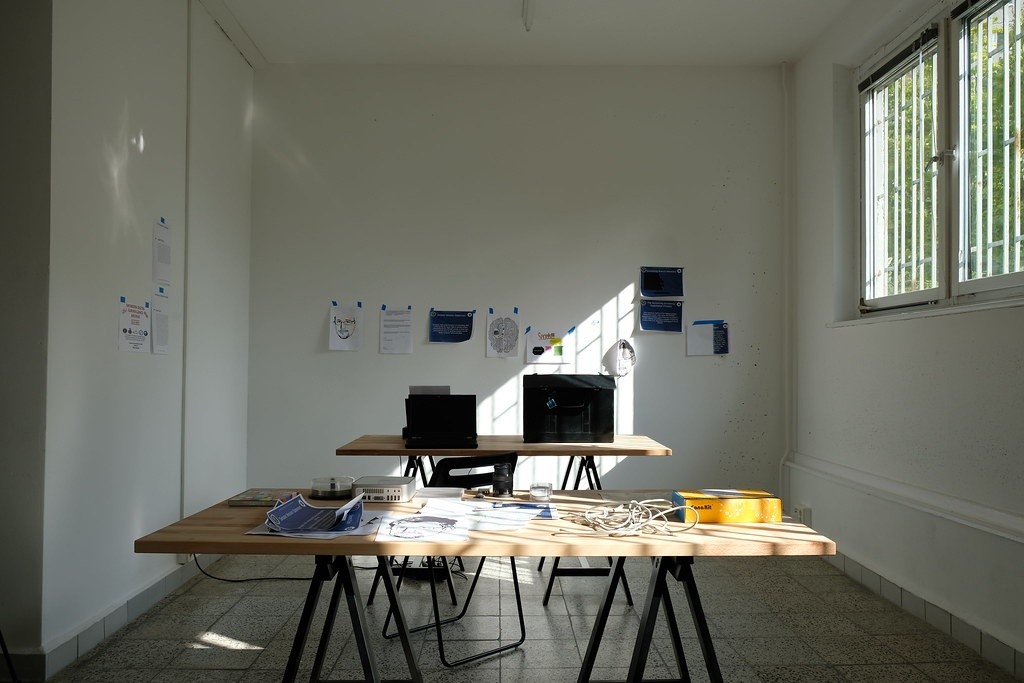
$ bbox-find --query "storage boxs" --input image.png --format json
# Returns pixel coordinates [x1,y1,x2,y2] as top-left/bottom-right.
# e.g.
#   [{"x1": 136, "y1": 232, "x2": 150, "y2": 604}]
[{"x1": 673, "y1": 489, "x2": 782, "y2": 523}]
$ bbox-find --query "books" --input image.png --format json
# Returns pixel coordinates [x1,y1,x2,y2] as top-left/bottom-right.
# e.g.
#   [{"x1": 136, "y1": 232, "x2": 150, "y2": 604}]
[{"x1": 228, "y1": 489, "x2": 302, "y2": 506}]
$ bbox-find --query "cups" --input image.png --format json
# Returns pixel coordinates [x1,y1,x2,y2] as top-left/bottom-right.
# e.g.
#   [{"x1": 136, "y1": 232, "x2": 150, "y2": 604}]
[{"x1": 494, "y1": 463, "x2": 513, "y2": 496}]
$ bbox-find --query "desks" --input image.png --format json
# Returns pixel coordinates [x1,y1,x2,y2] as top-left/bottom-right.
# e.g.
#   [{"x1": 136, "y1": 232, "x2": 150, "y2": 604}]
[
  {"x1": 135, "y1": 489, "x2": 836, "y2": 683},
  {"x1": 335, "y1": 435, "x2": 673, "y2": 605}
]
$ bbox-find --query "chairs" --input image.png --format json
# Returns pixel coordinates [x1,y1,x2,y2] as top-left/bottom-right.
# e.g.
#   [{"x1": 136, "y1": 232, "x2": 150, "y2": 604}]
[{"x1": 383, "y1": 453, "x2": 526, "y2": 668}]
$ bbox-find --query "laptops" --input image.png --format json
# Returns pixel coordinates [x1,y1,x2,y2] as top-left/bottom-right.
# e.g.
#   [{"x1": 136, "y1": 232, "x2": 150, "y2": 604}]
[{"x1": 404, "y1": 394, "x2": 477, "y2": 450}]
